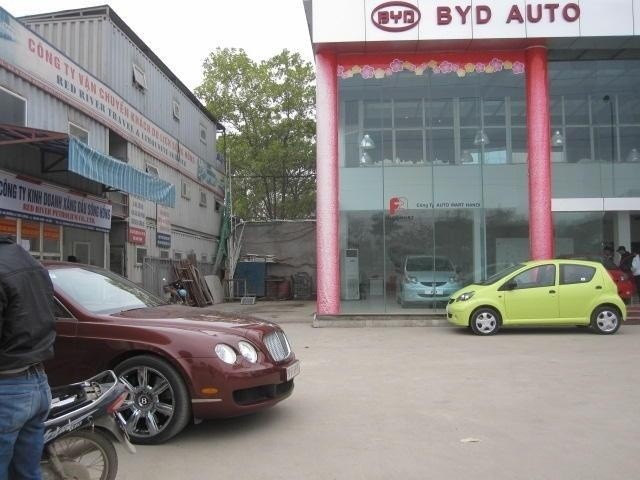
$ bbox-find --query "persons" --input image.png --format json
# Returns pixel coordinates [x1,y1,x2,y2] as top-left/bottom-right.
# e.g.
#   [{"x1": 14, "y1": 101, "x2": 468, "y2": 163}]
[
  {"x1": 603, "y1": 246, "x2": 615, "y2": 270},
  {"x1": 616, "y1": 245, "x2": 634, "y2": 272},
  {"x1": 628, "y1": 246, "x2": 640, "y2": 294},
  {"x1": 0, "y1": 225, "x2": 62, "y2": 479}
]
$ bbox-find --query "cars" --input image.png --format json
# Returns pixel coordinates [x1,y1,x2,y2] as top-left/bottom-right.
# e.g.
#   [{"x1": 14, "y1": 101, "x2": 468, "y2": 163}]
[
  {"x1": 444, "y1": 258, "x2": 628, "y2": 337},
  {"x1": 558, "y1": 254, "x2": 634, "y2": 305},
  {"x1": 396, "y1": 254, "x2": 463, "y2": 308},
  {"x1": 37, "y1": 258, "x2": 302, "y2": 446}
]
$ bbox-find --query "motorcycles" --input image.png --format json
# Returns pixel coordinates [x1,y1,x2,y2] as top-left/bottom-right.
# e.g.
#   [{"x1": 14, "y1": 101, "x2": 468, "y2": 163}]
[{"x1": 39, "y1": 369, "x2": 138, "y2": 480}]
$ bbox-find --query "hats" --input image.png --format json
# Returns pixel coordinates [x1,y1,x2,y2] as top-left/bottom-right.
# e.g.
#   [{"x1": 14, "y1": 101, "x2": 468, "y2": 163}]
[{"x1": 616, "y1": 246, "x2": 625, "y2": 252}]
